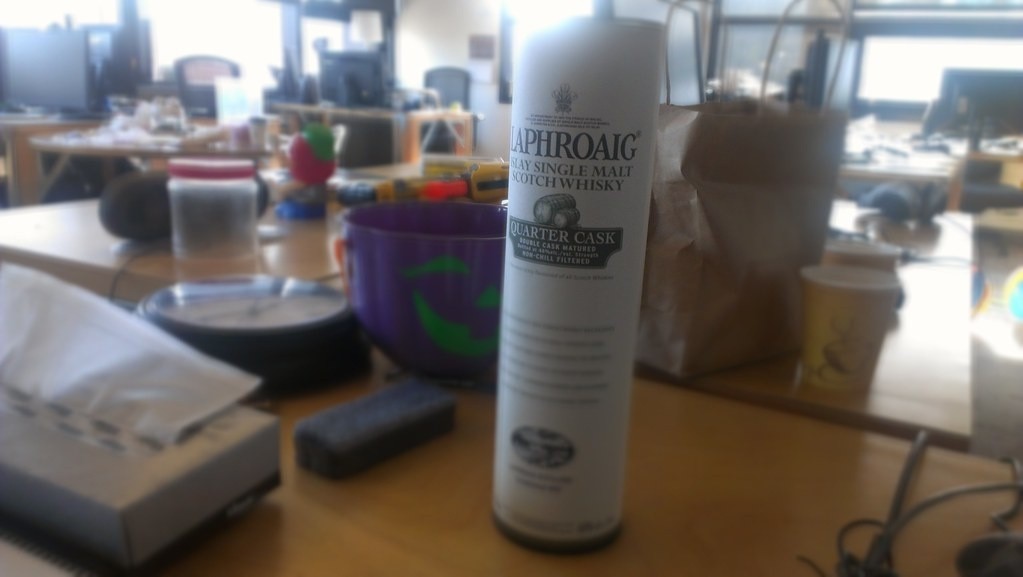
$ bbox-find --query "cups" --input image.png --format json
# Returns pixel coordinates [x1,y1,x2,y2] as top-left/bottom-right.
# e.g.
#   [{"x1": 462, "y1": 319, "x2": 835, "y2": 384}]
[{"x1": 798, "y1": 264, "x2": 903, "y2": 389}]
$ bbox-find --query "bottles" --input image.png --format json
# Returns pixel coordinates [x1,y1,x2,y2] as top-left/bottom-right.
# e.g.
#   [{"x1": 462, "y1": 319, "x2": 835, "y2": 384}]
[{"x1": 166, "y1": 157, "x2": 258, "y2": 258}]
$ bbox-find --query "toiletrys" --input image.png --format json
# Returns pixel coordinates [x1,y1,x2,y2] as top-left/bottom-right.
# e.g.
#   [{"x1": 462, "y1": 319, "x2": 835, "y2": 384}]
[{"x1": 491, "y1": 18, "x2": 672, "y2": 552}]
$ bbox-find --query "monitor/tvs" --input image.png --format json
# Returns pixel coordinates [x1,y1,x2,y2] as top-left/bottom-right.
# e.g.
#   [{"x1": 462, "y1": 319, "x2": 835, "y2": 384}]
[
  {"x1": 320, "y1": 51, "x2": 387, "y2": 109},
  {"x1": 4, "y1": 20, "x2": 151, "y2": 116},
  {"x1": 935, "y1": 70, "x2": 1023, "y2": 152}
]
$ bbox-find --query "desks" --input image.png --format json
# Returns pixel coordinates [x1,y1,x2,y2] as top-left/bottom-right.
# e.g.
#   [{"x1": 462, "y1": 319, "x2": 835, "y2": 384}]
[{"x1": 0, "y1": 113, "x2": 1023, "y2": 577}]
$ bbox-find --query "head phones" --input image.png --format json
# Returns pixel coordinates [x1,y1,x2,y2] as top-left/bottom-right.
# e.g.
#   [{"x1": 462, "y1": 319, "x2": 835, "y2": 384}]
[{"x1": 857, "y1": 181, "x2": 948, "y2": 224}]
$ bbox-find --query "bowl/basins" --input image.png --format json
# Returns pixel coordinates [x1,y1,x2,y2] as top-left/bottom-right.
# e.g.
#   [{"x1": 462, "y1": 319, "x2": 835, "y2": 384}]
[{"x1": 335, "y1": 200, "x2": 508, "y2": 381}]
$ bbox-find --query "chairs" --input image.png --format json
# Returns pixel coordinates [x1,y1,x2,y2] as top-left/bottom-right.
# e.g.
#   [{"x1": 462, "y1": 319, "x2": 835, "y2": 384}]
[{"x1": 417, "y1": 69, "x2": 478, "y2": 152}]
